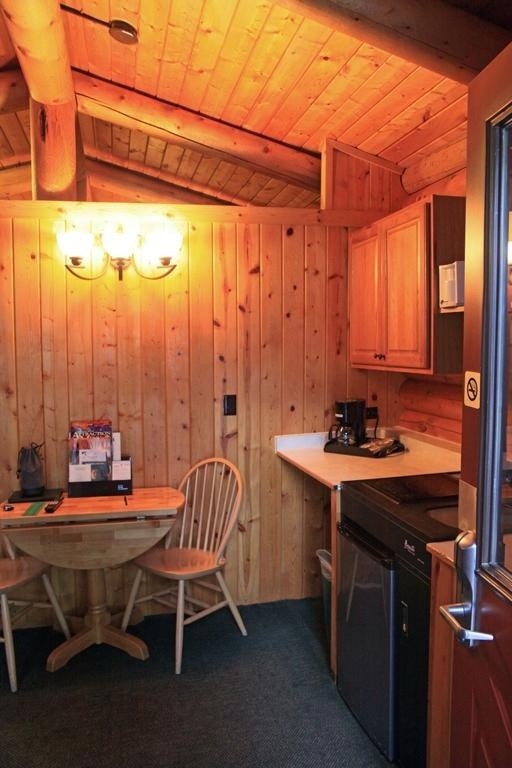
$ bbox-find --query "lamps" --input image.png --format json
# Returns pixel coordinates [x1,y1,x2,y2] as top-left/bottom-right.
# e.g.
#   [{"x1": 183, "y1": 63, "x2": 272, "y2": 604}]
[{"x1": 48, "y1": 214, "x2": 182, "y2": 282}]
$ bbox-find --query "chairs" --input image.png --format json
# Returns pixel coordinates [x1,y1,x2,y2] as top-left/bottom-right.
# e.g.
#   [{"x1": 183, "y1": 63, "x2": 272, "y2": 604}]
[
  {"x1": 0, "y1": 532, "x2": 72, "y2": 693},
  {"x1": 120, "y1": 457, "x2": 248, "y2": 675}
]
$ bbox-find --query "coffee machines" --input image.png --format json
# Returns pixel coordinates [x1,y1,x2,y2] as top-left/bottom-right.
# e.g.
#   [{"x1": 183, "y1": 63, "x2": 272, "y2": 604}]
[{"x1": 323, "y1": 395, "x2": 405, "y2": 458}]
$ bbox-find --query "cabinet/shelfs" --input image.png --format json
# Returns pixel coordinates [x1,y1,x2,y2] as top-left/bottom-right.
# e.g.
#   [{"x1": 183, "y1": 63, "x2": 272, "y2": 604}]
[{"x1": 345, "y1": 192, "x2": 467, "y2": 376}]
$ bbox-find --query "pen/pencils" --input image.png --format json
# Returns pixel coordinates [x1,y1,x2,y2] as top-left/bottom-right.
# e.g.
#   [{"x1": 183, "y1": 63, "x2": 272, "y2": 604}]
[{"x1": 123, "y1": 495, "x2": 129, "y2": 509}]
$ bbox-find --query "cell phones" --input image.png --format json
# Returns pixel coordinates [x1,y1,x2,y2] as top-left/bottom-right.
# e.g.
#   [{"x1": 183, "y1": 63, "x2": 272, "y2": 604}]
[{"x1": 45, "y1": 497, "x2": 64, "y2": 513}]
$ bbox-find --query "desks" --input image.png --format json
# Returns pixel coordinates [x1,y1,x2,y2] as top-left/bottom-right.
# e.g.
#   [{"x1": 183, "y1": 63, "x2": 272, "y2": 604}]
[
  {"x1": 276, "y1": 441, "x2": 460, "y2": 682},
  {"x1": 1, "y1": 488, "x2": 186, "y2": 673}
]
